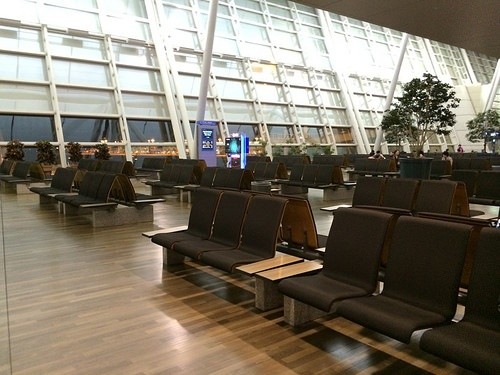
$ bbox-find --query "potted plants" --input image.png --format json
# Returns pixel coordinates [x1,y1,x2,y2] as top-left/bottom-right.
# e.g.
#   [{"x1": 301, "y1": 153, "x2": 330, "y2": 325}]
[
  {"x1": 380, "y1": 73, "x2": 460, "y2": 180},
  {"x1": 67, "y1": 140, "x2": 83, "y2": 168},
  {"x1": 35, "y1": 139, "x2": 58, "y2": 175}
]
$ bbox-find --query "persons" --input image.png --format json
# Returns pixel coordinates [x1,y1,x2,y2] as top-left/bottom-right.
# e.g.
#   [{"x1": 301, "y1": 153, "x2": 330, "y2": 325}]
[{"x1": 367, "y1": 144, "x2": 464, "y2": 167}]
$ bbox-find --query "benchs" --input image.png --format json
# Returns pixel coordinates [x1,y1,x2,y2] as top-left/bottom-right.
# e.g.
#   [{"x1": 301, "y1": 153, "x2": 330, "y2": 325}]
[{"x1": 0, "y1": 153, "x2": 500, "y2": 375}]
